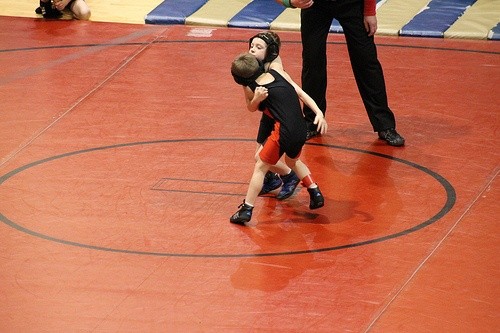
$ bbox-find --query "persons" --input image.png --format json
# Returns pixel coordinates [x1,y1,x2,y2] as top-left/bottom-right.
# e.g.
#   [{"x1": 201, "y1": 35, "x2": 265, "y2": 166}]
[
  {"x1": 277, "y1": 0, "x2": 405, "y2": 147},
  {"x1": 36, "y1": 0, "x2": 91, "y2": 20},
  {"x1": 229, "y1": 53, "x2": 324, "y2": 223},
  {"x1": 243, "y1": 31, "x2": 329, "y2": 200}
]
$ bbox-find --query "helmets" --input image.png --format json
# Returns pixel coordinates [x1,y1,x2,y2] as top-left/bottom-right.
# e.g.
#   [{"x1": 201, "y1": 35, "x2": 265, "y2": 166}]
[
  {"x1": 249, "y1": 32, "x2": 279, "y2": 62},
  {"x1": 232, "y1": 58, "x2": 264, "y2": 87}
]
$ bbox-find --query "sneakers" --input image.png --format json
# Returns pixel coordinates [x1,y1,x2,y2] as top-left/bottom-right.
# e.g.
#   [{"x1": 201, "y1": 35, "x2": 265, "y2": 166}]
[
  {"x1": 258, "y1": 169, "x2": 300, "y2": 200},
  {"x1": 378, "y1": 127, "x2": 405, "y2": 147},
  {"x1": 229, "y1": 199, "x2": 253, "y2": 223},
  {"x1": 305, "y1": 128, "x2": 320, "y2": 140},
  {"x1": 306, "y1": 183, "x2": 325, "y2": 210}
]
